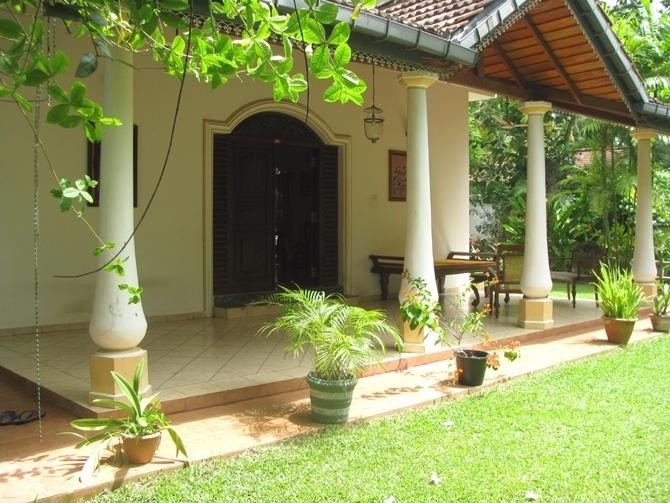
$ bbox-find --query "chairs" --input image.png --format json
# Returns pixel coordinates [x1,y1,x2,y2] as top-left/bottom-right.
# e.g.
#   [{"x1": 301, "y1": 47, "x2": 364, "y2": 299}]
[
  {"x1": 490, "y1": 244, "x2": 524, "y2": 309},
  {"x1": 546, "y1": 241, "x2": 602, "y2": 308}
]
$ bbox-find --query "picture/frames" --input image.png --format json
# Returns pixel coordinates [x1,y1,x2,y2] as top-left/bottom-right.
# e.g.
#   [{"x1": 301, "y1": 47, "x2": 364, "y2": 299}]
[{"x1": 389, "y1": 149, "x2": 406, "y2": 201}]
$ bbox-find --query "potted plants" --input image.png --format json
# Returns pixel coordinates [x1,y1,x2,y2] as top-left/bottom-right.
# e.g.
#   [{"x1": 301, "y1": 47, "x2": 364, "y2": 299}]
[
  {"x1": 648, "y1": 276, "x2": 670, "y2": 332},
  {"x1": 53, "y1": 354, "x2": 189, "y2": 485},
  {"x1": 588, "y1": 257, "x2": 647, "y2": 344},
  {"x1": 242, "y1": 277, "x2": 406, "y2": 425},
  {"x1": 398, "y1": 269, "x2": 522, "y2": 386}
]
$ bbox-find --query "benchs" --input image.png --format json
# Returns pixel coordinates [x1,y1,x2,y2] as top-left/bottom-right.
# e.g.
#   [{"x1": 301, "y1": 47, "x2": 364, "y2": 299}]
[{"x1": 369, "y1": 251, "x2": 500, "y2": 300}]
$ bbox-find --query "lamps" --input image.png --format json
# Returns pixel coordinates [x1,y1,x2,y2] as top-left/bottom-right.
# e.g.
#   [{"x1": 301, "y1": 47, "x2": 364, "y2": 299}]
[{"x1": 362, "y1": 64, "x2": 384, "y2": 144}]
